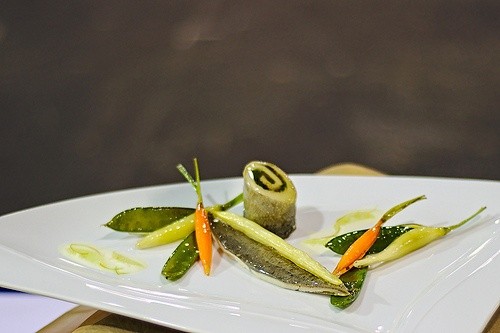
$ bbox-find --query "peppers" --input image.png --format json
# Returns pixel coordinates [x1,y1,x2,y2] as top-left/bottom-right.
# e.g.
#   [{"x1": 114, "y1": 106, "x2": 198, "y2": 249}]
[
  {"x1": 331, "y1": 194, "x2": 427, "y2": 275},
  {"x1": 353, "y1": 205, "x2": 488, "y2": 269},
  {"x1": 193, "y1": 157, "x2": 212, "y2": 276},
  {"x1": 136, "y1": 163, "x2": 205, "y2": 248}
]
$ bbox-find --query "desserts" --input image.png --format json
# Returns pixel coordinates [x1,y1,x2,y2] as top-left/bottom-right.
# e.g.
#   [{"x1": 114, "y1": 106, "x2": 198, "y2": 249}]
[{"x1": 242, "y1": 160, "x2": 296, "y2": 239}]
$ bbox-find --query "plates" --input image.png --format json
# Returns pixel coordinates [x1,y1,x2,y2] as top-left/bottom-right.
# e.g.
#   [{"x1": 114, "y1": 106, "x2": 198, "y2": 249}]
[{"x1": 0, "y1": 175, "x2": 499, "y2": 332}]
